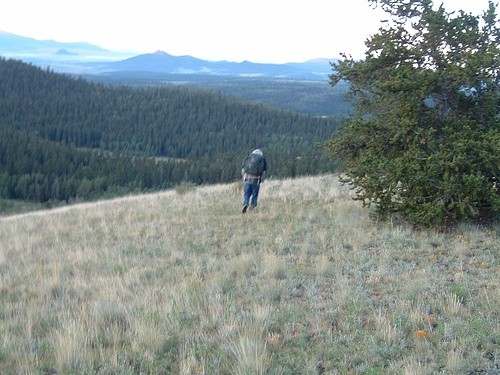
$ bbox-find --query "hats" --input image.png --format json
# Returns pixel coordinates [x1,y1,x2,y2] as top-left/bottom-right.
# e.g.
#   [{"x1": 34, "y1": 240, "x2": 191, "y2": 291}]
[{"x1": 252, "y1": 149, "x2": 263, "y2": 156}]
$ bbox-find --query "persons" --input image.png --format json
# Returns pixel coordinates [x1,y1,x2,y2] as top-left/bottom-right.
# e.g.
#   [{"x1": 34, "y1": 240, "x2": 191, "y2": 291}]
[{"x1": 238, "y1": 148, "x2": 267, "y2": 213}]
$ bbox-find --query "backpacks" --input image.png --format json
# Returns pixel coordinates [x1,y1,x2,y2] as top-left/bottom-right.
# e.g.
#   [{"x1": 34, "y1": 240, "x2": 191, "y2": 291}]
[{"x1": 243, "y1": 153, "x2": 266, "y2": 176}]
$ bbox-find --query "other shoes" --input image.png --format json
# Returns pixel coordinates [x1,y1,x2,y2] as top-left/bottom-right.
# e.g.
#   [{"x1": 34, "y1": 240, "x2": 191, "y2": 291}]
[
  {"x1": 250, "y1": 206, "x2": 257, "y2": 210},
  {"x1": 241, "y1": 204, "x2": 247, "y2": 213}
]
